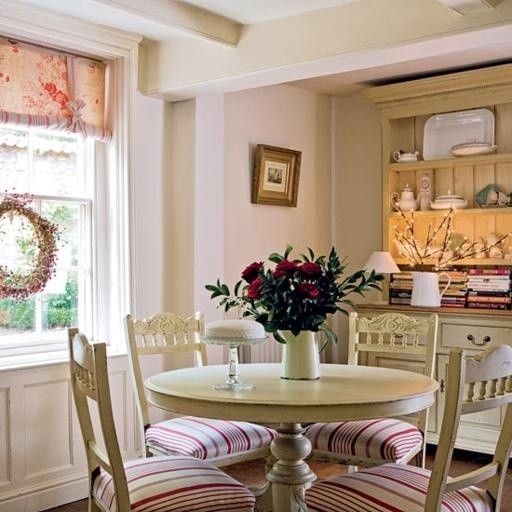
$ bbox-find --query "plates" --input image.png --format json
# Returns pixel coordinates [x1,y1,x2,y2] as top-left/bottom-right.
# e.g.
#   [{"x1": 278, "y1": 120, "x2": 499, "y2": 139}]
[
  {"x1": 421, "y1": 108, "x2": 495, "y2": 160},
  {"x1": 449, "y1": 143, "x2": 497, "y2": 157}
]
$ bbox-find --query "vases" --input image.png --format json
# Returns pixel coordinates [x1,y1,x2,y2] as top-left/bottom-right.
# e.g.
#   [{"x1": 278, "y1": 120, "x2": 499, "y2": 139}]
[{"x1": 278, "y1": 328, "x2": 323, "y2": 380}]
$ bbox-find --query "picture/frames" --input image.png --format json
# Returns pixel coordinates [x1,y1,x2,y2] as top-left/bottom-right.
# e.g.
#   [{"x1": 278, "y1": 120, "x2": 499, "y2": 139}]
[{"x1": 250, "y1": 142, "x2": 302, "y2": 208}]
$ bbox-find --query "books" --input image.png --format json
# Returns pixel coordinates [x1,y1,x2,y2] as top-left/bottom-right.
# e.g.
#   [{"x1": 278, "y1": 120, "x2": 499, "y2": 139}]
[{"x1": 390, "y1": 269, "x2": 511, "y2": 309}]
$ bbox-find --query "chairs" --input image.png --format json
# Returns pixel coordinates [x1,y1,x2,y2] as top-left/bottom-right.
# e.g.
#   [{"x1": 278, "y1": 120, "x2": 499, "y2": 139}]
[
  {"x1": 301, "y1": 306, "x2": 441, "y2": 471},
  {"x1": 61, "y1": 318, "x2": 259, "y2": 512},
  {"x1": 124, "y1": 308, "x2": 280, "y2": 481},
  {"x1": 298, "y1": 341, "x2": 512, "y2": 512}
]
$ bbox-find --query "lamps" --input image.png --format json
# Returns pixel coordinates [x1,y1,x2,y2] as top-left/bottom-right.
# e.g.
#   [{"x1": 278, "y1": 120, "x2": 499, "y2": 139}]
[{"x1": 360, "y1": 251, "x2": 401, "y2": 305}]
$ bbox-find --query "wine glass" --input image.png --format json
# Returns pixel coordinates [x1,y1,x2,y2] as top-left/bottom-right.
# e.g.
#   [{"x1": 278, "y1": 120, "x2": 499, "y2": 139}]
[{"x1": 200, "y1": 335, "x2": 270, "y2": 391}]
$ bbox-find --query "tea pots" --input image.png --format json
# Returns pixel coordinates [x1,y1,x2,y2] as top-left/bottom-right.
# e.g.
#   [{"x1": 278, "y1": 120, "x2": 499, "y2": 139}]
[
  {"x1": 429, "y1": 190, "x2": 470, "y2": 210},
  {"x1": 392, "y1": 150, "x2": 419, "y2": 162},
  {"x1": 275, "y1": 329, "x2": 329, "y2": 381},
  {"x1": 391, "y1": 183, "x2": 420, "y2": 212}
]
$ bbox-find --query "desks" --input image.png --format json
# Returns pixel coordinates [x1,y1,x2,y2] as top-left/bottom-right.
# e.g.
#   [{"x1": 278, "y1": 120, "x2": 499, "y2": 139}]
[{"x1": 145, "y1": 360, "x2": 441, "y2": 509}]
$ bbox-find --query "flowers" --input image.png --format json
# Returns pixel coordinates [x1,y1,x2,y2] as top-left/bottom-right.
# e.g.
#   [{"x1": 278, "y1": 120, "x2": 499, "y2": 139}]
[
  {"x1": 0, "y1": 186, "x2": 60, "y2": 301},
  {"x1": 203, "y1": 242, "x2": 385, "y2": 354},
  {"x1": 388, "y1": 198, "x2": 512, "y2": 272}
]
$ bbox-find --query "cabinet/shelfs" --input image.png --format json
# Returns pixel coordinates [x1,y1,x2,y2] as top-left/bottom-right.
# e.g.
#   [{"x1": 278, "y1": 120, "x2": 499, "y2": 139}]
[
  {"x1": 434, "y1": 305, "x2": 512, "y2": 461},
  {"x1": 357, "y1": 60, "x2": 512, "y2": 274},
  {"x1": 348, "y1": 301, "x2": 438, "y2": 447}
]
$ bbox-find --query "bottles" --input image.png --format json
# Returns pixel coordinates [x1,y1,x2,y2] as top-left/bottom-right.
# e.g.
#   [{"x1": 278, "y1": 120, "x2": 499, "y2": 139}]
[
  {"x1": 457, "y1": 233, "x2": 503, "y2": 260},
  {"x1": 420, "y1": 170, "x2": 434, "y2": 210}
]
